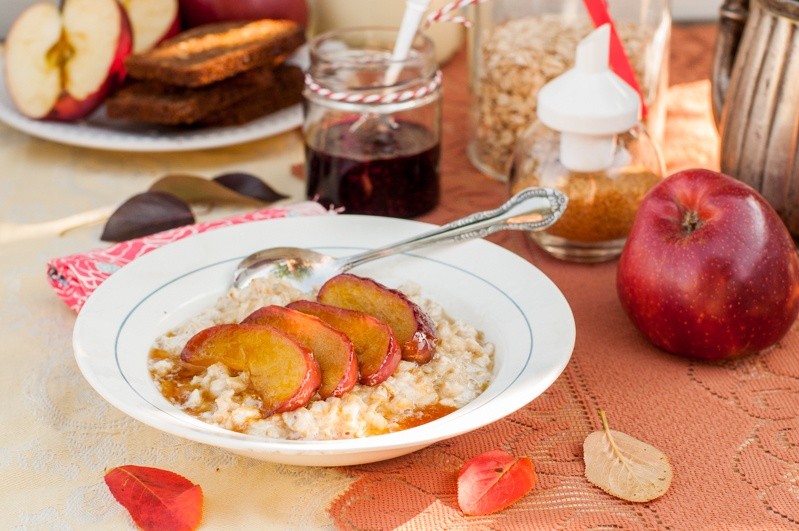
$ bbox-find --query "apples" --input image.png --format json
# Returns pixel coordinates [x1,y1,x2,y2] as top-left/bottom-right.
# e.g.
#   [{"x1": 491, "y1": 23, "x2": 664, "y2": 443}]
[
  {"x1": 613, "y1": 167, "x2": 798, "y2": 364},
  {"x1": 0, "y1": 2, "x2": 183, "y2": 133}
]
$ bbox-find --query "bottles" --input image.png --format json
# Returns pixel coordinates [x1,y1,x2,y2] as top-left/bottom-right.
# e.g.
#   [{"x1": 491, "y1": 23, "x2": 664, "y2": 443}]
[
  {"x1": 302, "y1": 29, "x2": 444, "y2": 219},
  {"x1": 508, "y1": 25, "x2": 667, "y2": 267}
]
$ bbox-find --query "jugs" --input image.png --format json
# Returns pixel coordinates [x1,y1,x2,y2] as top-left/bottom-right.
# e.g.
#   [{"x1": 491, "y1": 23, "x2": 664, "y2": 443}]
[{"x1": 710, "y1": 0, "x2": 799, "y2": 254}]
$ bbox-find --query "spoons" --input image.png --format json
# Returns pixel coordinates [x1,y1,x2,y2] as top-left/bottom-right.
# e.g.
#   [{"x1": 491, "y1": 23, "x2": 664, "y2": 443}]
[{"x1": 233, "y1": 185, "x2": 568, "y2": 303}]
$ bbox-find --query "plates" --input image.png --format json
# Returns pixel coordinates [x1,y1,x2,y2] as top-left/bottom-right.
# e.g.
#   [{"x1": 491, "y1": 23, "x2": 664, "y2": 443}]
[
  {"x1": 1, "y1": 39, "x2": 351, "y2": 155},
  {"x1": 69, "y1": 215, "x2": 579, "y2": 470}
]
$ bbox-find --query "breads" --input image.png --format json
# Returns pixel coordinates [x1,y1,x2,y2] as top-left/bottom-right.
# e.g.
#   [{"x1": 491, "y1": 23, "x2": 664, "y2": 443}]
[
  {"x1": 105, "y1": 65, "x2": 279, "y2": 126},
  {"x1": 207, "y1": 84, "x2": 308, "y2": 124},
  {"x1": 118, "y1": 15, "x2": 304, "y2": 87}
]
